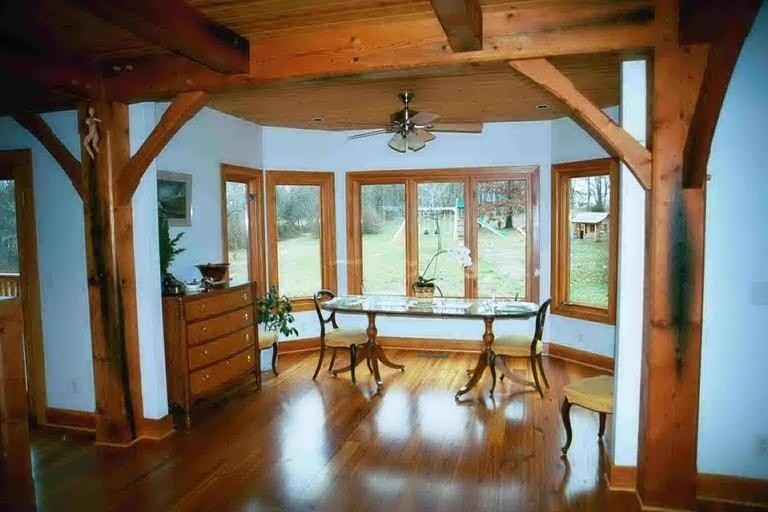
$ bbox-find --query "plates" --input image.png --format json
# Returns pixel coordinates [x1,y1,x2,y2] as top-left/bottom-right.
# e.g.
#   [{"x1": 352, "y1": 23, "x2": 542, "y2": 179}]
[
  {"x1": 181, "y1": 288, "x2": 206, "y2": 294},
  {"x1": 198, "y1": 278, "x2": 234, "y2": 287}
]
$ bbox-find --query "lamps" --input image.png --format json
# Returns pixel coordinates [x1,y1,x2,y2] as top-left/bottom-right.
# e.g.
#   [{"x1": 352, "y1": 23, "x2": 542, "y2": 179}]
[{"x1": 386, "y1": 90, "x2": 437, "y2": 151}]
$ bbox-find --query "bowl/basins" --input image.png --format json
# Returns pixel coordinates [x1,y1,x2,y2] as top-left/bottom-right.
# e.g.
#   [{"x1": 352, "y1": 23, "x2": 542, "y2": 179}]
[
  {"x1": 194, "y1": 263, "x2": 230, "y2": 282},
  {"x1": 184, "y1": 282, "x2": 201, "y2": 290}
]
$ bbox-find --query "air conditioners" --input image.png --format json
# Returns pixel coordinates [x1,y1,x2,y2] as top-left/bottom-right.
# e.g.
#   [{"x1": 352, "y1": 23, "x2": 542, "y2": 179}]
[{"x1": 337, "y1": 110, "x2": 485, "y2": 141}]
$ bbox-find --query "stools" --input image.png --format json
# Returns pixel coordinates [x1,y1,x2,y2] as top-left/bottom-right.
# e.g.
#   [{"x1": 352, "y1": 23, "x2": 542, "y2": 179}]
[
  {"x1": 561, "y1": 369, "x2": 614, "y2": 454},
  {"x1": 258, "y1": 331, "x2": 281, "y2": 378}
]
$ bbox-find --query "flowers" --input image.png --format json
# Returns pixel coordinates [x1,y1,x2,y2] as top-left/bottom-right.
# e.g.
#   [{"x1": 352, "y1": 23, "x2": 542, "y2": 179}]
[{"x1": 413, "y1": 244, "x2": 473, "y2": 286}]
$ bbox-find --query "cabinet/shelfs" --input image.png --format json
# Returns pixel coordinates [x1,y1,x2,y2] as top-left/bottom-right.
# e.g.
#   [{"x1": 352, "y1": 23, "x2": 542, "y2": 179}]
[{"x1": 165, "y1": 282, "x2": 253, "y2": 434}]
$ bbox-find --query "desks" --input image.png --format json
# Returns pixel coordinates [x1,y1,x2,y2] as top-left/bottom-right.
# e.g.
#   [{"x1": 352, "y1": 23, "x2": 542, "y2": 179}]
[{"x1": 321, "y1": 294, "x2": 541, "y2": 402}]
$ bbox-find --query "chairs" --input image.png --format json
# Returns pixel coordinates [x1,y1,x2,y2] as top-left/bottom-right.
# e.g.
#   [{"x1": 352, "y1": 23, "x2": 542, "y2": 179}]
[
  {"x1": 308, "y1": 287, "x2": 372, "y2": 385},
  {"x1": 488, "y1": 297, "x2": 552, "y2": 399}
]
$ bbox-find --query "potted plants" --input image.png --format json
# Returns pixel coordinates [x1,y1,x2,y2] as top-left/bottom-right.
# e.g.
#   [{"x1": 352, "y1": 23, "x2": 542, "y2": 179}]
[{"x1": 257, "y1": 284, "x2": 298, "y2": 372}]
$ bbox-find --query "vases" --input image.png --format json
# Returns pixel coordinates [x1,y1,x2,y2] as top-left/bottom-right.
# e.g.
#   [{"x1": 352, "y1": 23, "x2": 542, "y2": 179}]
[{"x1": 412, "y1": 285, "x2": 434, "y2": 303}]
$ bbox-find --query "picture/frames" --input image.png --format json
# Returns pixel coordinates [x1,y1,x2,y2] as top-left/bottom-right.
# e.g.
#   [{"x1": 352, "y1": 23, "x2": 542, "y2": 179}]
[{"x1": 156, "y1": 170, "x2": 193, "y2": 227}]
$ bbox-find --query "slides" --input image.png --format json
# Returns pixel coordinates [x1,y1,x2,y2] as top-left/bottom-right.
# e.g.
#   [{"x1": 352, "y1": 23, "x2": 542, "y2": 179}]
[{"x1": 477, "y1": 216, "x2": 507, "y2": 238}]
